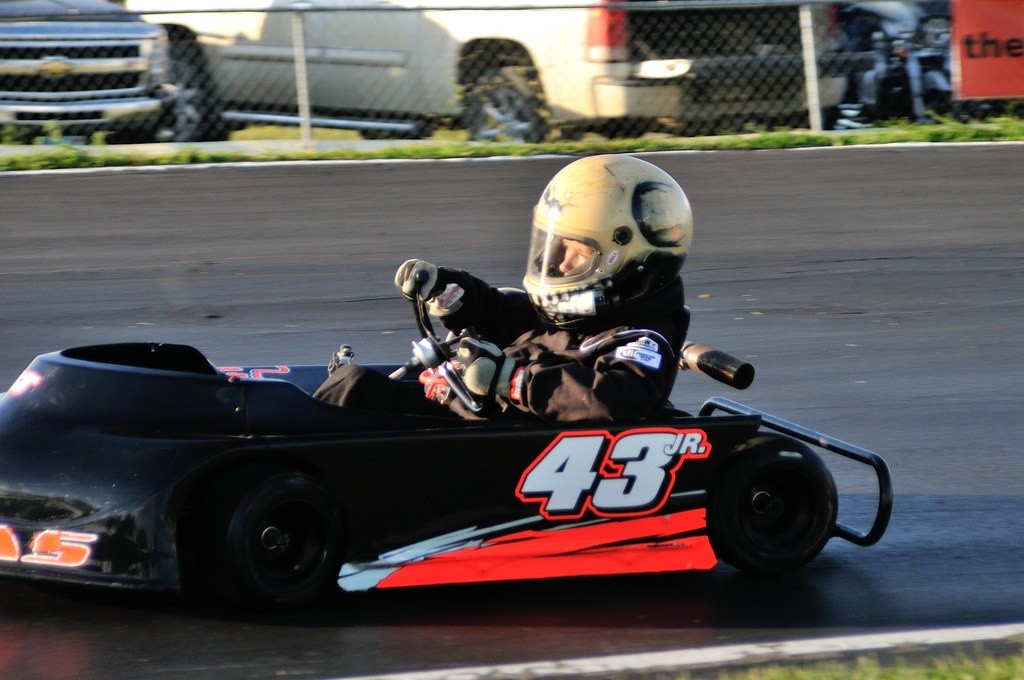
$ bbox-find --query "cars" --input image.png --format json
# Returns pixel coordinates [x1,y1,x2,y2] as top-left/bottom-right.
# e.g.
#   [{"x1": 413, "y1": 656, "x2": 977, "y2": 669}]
[{"x1": 0, "y1": 0, "x2": 179, "y2": 146}]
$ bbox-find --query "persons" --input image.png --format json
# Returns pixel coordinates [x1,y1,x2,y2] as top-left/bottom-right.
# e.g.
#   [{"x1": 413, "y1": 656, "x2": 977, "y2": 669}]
[{"x1": 312, "y1": 154, "x2": 694, "y2": 423}]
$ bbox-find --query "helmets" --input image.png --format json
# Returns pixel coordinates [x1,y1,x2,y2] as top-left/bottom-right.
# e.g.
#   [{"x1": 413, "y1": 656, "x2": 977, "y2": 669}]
[{"x1": 524, "y1": 154, "x2": 694, "y2": 331}]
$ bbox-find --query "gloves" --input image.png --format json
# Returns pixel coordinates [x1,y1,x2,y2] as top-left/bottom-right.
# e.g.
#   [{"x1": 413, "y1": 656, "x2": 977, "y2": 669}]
[
  {"x1": 394, "y1": 258, "x2": 454, "y2": 304},
  {"x1": 456, "y1": 336, "x2": 516, "y2": 396}
]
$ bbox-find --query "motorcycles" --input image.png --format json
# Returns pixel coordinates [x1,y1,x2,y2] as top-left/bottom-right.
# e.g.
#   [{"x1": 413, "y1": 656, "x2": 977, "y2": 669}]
[{"x1": 842, "y1": 4, "x2": 962, "y2": 124}]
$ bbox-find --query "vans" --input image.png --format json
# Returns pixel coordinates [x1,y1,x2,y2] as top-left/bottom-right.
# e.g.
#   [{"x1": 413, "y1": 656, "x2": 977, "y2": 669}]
[{"x1": 137, "y1": 0, "x2": 855, "y2": 145}]
